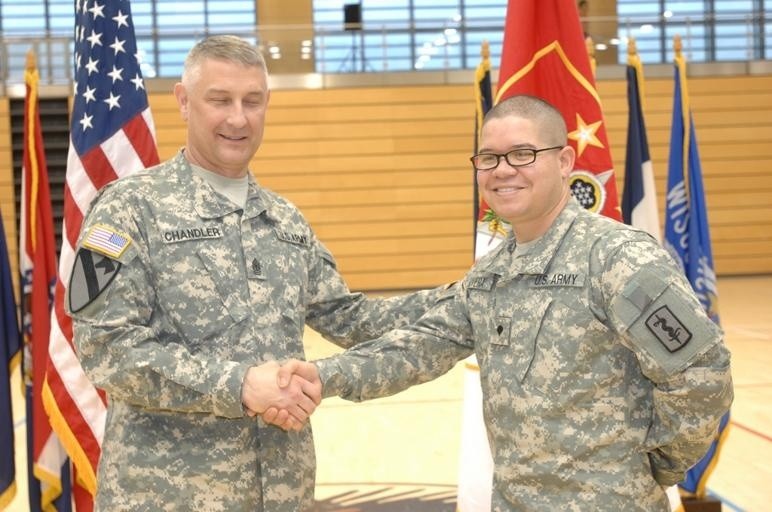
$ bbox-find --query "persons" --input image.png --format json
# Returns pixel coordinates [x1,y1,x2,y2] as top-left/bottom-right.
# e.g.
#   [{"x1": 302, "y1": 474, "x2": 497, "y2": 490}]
[
  {"x1": 64, "y1": 34, "x2": 460, "y2": 512},
  {"x1": 248, "y1": 96, "x2": 734, "y2": 512}
]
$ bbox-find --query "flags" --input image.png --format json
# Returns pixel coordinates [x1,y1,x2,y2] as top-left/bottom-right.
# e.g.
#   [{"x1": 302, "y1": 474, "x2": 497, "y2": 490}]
[
  {"x1": 1, "y1": 1, "x2": 162, "y2": 512},
  {"x1": 458, "y1": 2, "x2": 730, "y2": 512}
]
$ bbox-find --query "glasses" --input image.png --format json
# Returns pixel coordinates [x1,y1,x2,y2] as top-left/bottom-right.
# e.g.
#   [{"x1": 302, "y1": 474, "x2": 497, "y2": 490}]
[{"x1": 470, "y1": 146, "x2": 563, "y2": 170}]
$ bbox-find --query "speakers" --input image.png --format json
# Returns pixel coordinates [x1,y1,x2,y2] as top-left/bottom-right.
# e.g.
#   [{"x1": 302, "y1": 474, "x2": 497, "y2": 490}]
[{"x1": 344, "y1": 4, "x2": 361, "y2": 30}]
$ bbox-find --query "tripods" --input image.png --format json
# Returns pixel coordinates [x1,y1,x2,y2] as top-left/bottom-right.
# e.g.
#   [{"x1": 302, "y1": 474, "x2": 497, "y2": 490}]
[{"x1": 338, "y1": 33, "x2": 372, "y2": 72}]
